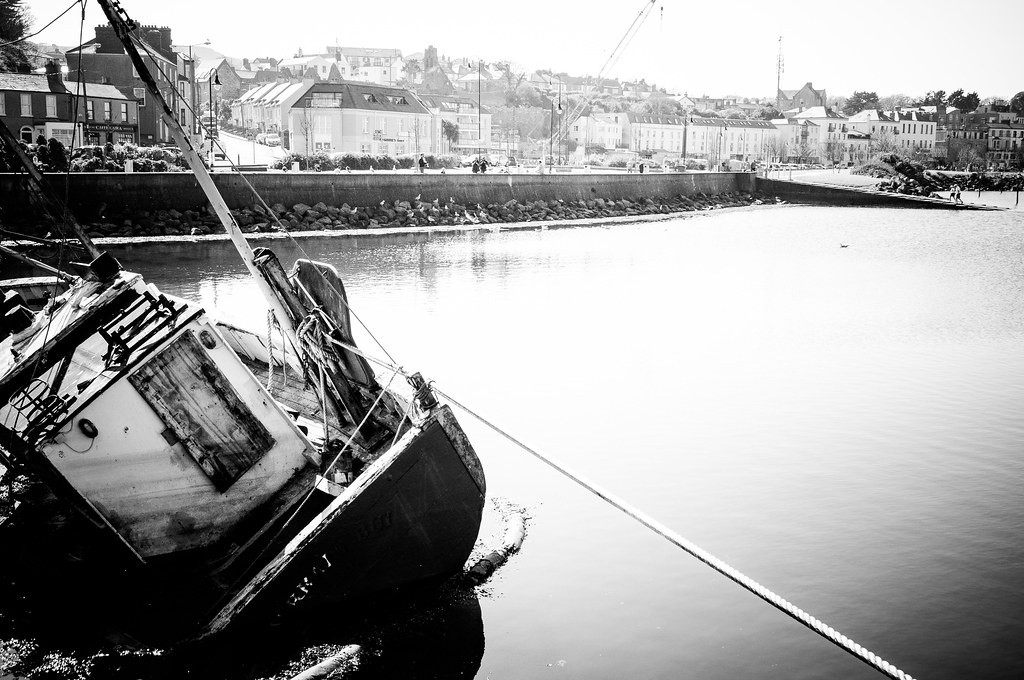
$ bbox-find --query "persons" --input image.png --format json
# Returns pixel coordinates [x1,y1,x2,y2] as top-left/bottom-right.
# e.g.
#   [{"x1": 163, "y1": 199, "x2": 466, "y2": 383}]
[
  {"x1": 627, "y1": 158, "x2": 648, "y2": 173},
  {"x1": 949, "y1": 184, "x2": 964, "y2": 204},
  {"x1": 721, "y1": 159, "x2": 728, "y2": 172},
  {"x1": 419, "y1": 154, "x2": 428, "y2": 173},
  {"x1": 471, "y1": 157, "x2": 490, "y2": 173},
  {"x1": 751, "y1": 159, "x2": 757, "y2": 172}
]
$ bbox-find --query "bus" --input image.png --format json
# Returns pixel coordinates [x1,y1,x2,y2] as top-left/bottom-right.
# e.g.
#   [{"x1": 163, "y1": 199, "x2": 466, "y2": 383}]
[{"x1": 200, "y1": 114, "x2": 218, "y2": 129}]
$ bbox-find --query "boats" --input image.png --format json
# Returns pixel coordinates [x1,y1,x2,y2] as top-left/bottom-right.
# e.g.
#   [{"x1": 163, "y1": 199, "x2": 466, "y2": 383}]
[{"x1": 0, "y1": 0, "x2": 487, "y2": 680}]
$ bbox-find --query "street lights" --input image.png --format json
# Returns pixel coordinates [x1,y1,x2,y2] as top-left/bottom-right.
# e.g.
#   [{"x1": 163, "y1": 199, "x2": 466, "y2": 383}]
[
  {"x1": 549, "y1": 98, "x2": 563, "y2": 174},
  {"x1": 549, "y1": 75, "x2": 561, "y2": 164},
  {"x1": 169, "y1": 42, "x2": 212, "y2": 142},
  {"x1": 683, "y1": 113, "x2": 693, "y2": 165},
  {"x1": 210, "y1": 68, "x2": 222, "y2": 172},
  {"x1": 467, "y1": 56, "x2": 481, "y2": 163}
]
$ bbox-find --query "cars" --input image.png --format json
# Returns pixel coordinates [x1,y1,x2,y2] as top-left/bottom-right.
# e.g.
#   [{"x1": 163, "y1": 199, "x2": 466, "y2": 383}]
[
  {"x1": 162, "y1": 147, "x2": 183, "y2": 162},
  {"x1": 766, "y1": 163, "x2": 827, "y2": 171},
  {"x1": 462, "y1": 153, "x2": 517, "y2": 167},
  {"x1": 256, "y1": 133, "x2": 268, "y2": 144},
  {"x1": 204, "y1": 127, "x2": 219, "y2": 141},
  {"x1": 264, "y1": 134, "x2": 281, "y2": 147}
]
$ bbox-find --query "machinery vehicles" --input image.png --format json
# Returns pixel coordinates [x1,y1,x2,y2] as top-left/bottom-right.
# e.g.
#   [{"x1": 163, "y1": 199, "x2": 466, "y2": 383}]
[{"x1": 524, "y1": 0, "x2": 664, "y2": 165}]
